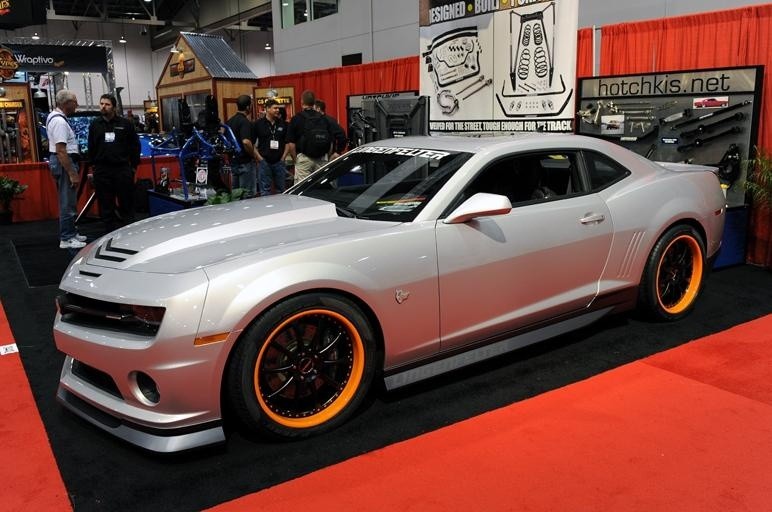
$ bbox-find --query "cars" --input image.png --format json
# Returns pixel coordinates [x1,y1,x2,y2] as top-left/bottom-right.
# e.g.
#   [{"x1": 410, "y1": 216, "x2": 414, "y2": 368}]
[{"x1": 694, "y1": 98, "x2": 728, "y2": 108}]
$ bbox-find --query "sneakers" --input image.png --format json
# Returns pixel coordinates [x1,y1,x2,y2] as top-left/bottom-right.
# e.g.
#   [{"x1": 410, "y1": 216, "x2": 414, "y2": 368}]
[{"x1": 59, "y1": 234, "x2": 87, "y2": 250}]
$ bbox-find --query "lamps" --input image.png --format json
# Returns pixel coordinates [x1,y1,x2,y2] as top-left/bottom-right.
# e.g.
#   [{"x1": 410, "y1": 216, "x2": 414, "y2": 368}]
[{"x1": 30, "y1": 1, "x2": 273, "y2": 52}]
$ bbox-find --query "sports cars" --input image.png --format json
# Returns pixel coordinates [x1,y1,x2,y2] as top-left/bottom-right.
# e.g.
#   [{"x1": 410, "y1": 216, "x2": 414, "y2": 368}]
[{"x1": 43, "y1": 131, "x2": 732, "y2": 457}]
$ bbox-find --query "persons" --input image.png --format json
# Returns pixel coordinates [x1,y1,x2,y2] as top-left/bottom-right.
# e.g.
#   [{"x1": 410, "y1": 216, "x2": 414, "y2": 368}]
[
  {"x1": 312, "y1": 99, "x2": 349, "y2": 190},
  {"x1": 133, "y1": 115, "x2": 146, "y2": 133},
  {"x1": 251, "y1": 99, "x2": 288, "y2": 195},
  {"x1": 88, "y1": 94, "x2": 142, "y2": 234},
  {"x1": 224, "y1": 95, "x2": 257, "y2": 200},
  {"x1": 45, "y1": 90, "x2": 89, "y2": 249},
  {"x1": 287, "y1": 90, "x2": 330, "y2": 185}
]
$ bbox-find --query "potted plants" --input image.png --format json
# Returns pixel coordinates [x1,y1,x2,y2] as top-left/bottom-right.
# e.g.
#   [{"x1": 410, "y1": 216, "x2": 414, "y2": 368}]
[{"x1": 2, "y1": 175, "x2": 28, "y2": 229}]
